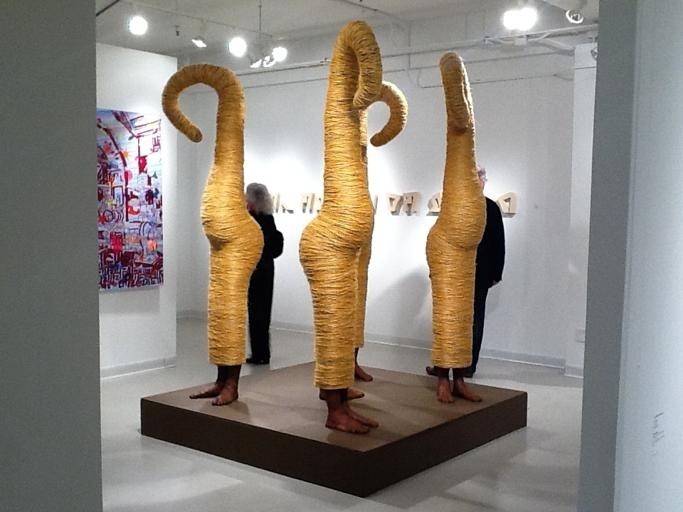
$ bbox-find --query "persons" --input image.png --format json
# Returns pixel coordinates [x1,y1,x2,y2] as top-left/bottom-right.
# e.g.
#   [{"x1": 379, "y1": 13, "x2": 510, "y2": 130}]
[
  {"x1": 317, "y1": 348, "x2": 373, "y2": 401},
  {"x1": 189, "y1": 364, "x2": 242, "y2": 406},
  {"x1": 425, "y1": 164, "x2": 507, "y2": 377},
  {"x1": 318, "y1": 389, "x2": 380, "y2": 435},
  {"x1": 436, "y1": 367, "x2": 482, "y2": 405},
  {"x1": 243, "y1": 183, "x2": 276, "y2": 366}
]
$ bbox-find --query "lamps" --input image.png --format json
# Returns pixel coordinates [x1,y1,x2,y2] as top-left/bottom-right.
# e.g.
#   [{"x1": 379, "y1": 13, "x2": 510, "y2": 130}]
[
  {"x1": 227, "y1": 1, "x2": 289, "y2": 68},
  {"x1": 501, "y1": 4, "x2": 542, "y2": 48},
  {"x1": 191, "y1": 19, "x2": 209, "y2": 48}
]
